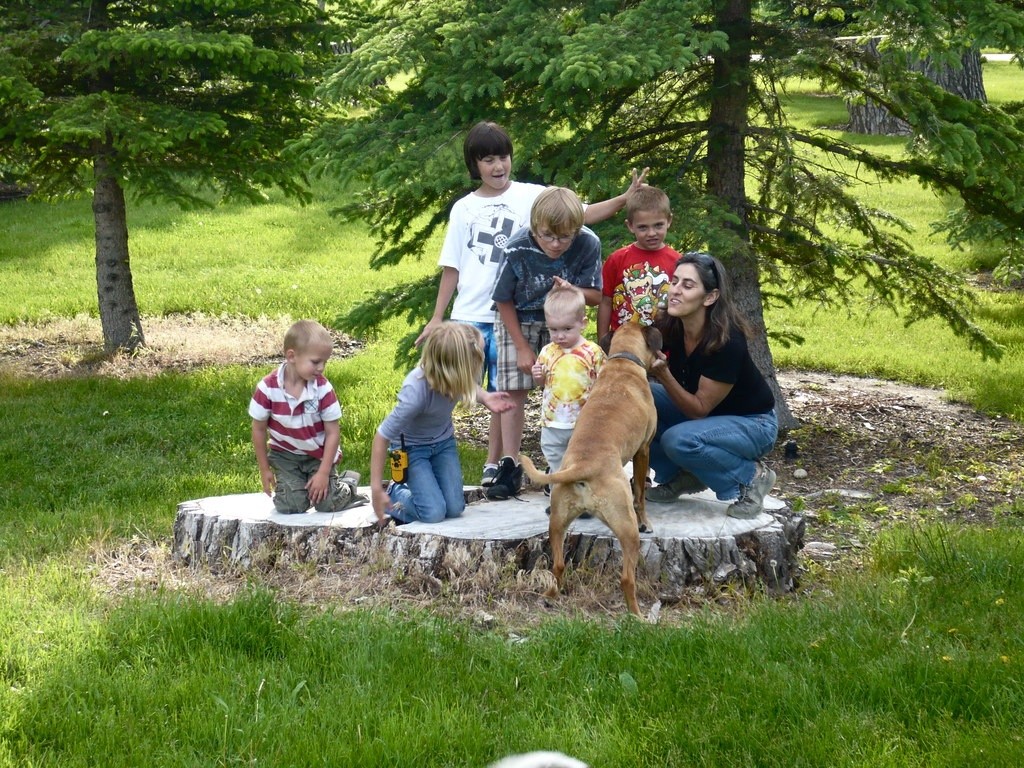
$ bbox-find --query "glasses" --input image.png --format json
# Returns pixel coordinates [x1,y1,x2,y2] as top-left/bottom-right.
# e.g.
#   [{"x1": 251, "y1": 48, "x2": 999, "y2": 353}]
[
  {"x1": 536, "y1": 231, "x2": 576, "y2": 244},
  {"x1": 687, "y1": 251, "x2": 719, "y2": 288}
]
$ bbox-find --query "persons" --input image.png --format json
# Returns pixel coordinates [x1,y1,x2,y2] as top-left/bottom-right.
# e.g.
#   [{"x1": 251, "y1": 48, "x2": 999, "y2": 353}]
[
  {"x1": 597, "y1": 187, "x2": 682, "y2": 487},
  {"x1": 248, "y1": 319, "x2": 369, "y2": 514},
  {"x1": 415, "y1": 122, "x2": 650, "y2": 490},
  {"x1": 371, "y1": 322, "x2": 515, "y2": 526},
  {"x1": 531, "y1": 287, "x2": 607, "y2": 518},
  {"x1": 486, "y1": 186, "x2": 602, "y2": 499},
  {"x1": 644, "y1": 254, "x2": 777, "y2": 519}
]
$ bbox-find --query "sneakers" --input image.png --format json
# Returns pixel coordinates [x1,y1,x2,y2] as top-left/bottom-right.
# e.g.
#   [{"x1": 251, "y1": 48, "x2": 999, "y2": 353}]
[
  {"x1": 645, "y1": 471, "x2": 708, "y2": 503},
  {"x1": 727, "y1": 458, "x2": 776, "y2": 519},
  {"x1": 485, "y1": 456, "x2": 524, "y2": 499}
]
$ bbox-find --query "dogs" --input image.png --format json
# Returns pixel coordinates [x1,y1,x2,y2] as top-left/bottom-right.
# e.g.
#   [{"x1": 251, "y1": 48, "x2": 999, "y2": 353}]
[{"x1": 520, "y1": 322, "x2": 663, "y2": 621}]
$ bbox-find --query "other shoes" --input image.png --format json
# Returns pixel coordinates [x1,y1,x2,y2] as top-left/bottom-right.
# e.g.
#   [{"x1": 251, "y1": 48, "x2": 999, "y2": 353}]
[
  {"x1": 630, "y1": 475, "x2": 652, "y2": 493},
  {"x1": 480, "y1": 463, "x2": 499, "y2": 485},
  {"x1": 339, "y1": 470, "x2": 361, "y2": 486},
  {"x1": 543, "y1": 466, "x2": 550, "y2": 496}
]
